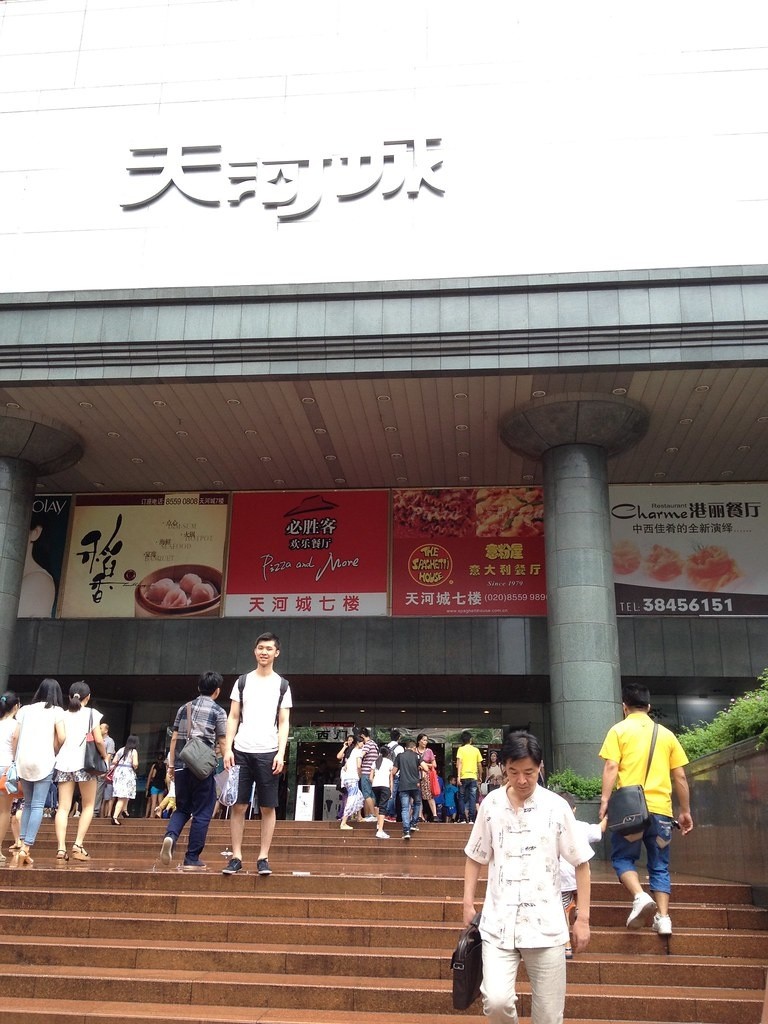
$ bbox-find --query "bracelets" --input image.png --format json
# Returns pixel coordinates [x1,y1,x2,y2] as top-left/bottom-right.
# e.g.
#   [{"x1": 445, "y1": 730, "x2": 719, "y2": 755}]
[{"x1": 168, "y1": 767, "x2": 175, "y2": 769}]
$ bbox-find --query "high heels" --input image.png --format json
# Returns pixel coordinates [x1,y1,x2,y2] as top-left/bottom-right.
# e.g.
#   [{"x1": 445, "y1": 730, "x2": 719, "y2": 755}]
[
  {"x1": 154, "y1": 806, "x2": 162, "y2": 819},
  {"x1": 72, "y1": 844, "x2": 90, "y2": 860},
  {"x1": 55, "y1": 850, "x2": 69, "y2": 864},
  {"x1": 18, "y1": 850, "x2": 34, "y2": 867}
]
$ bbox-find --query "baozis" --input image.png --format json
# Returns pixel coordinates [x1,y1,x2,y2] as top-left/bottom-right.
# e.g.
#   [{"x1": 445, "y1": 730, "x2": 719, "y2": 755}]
[{"x1": 147, "y1": 572, "x2": 215, "y2": 608}]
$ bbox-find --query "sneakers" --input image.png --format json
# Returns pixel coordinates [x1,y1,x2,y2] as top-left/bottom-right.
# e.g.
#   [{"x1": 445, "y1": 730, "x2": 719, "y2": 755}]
[
  {"x1": 256, "y1": 858, "x2": 273, "y2": 874},
  {"x1": 652, "y1": 912, "x2": 673, "y2": 935},
  {"x1": 222, "y1": 858, "x2": 243, "y2": 874},
  {"x1": 626, "y1": 893, "x2": 657, "y2": 930}
]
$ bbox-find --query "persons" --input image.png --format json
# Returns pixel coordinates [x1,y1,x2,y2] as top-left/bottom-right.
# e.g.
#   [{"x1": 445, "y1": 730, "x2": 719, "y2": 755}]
[
  {"x1": 221, "y1": 633, "x2": 293, "y2": 874},
  {"x1": 0, "y1": 678, "x2": 256, "y2": 864},
  {"x1": 463, "y1": 730, "x2": 595, "y2": 1024},
  {"x1": 159, "y1": 671, "x2": 227, "y2": 872},
  {"x1": 337, "y1": 726, "x2": 509, "y2": 839},
  {"x1": 557, "y1": 792, "x2": 608, "y2": 959},
  {"x1": 598, "y1": 683, "x2": 693, "y2": 934}
]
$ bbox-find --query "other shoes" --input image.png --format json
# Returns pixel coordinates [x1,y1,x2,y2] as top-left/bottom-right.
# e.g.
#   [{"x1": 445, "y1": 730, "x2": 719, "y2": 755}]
[
  {"x1": 335, "y1": 818, "x2": 474, "y2": 839},
  {"x1": 183, "y1": 863, "x2": 207, "y2": 871},
  {"x1": 160, "y1": 836, "x2": 173, "y2": 865}
]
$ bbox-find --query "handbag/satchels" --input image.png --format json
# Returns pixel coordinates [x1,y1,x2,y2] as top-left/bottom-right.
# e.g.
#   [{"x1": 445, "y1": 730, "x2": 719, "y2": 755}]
[
  {"x1": 450, "y1": 911, "x2": 484, "y2": 1010},
  {"x1": 0, "y1": 767, "x2": 25, "y2": 798},
  {"x1": 430, "y1": 765, "x2": 441, "y2": 797},
  {"x1": 85, "y1": 732, "x2": 107, "y2": 776},
  {"x1": 178, "y1": 737, "x2": 219, "y2": 781},
  {"x1": 608, "y1": 785, "x2": 648, "y2": 836},
  {"x1": 214, "y1": 765, "x2": 241, "y2": 806},
  {"x1": 480, "y1": 778, "x2": 500, "y2": 796},
  {"x1": 105, "y1": 766, "x2": 115, "y2": 784}
]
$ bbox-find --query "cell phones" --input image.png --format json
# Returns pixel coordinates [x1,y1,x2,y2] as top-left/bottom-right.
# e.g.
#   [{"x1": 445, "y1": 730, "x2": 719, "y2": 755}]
[{"x1": 346, "y1": 743, "x2": 348, "y2": 746}]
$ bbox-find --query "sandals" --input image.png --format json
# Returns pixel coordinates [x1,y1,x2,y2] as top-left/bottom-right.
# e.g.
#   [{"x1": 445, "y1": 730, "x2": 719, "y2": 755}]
[
  {"x1": 122, "y1": 810, "x2": 130, "y2": 818},
  {"x1": 111, "y1": 817, "x2": 121, "y2": 825},
  {"x1": 0, "y1": 852, "x2": 7, "y2": 862},
  {"x1": 565, "y1": 948, "x2": 572, "y2": 959}
]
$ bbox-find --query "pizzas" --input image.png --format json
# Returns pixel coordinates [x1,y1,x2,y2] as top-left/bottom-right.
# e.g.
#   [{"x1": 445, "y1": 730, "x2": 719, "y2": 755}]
[{"x1": 392, "y1": 487, "x2": 545, "y2": 537}]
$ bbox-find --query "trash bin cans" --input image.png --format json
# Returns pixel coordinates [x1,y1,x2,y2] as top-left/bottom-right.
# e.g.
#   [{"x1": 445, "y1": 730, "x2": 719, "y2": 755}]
[
  {"x1": 294, "y1": 785, "x2": 315, "y2": 821},
  {"x1": 322, "y1": 784, "x2": 342, "y2": 821}
]
